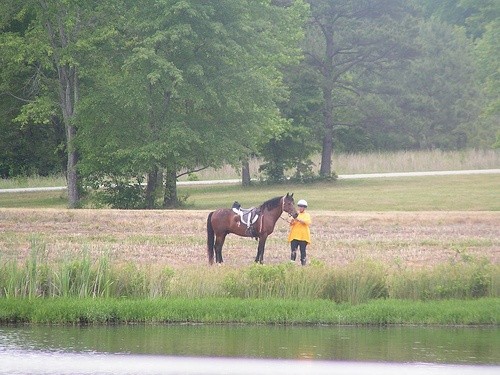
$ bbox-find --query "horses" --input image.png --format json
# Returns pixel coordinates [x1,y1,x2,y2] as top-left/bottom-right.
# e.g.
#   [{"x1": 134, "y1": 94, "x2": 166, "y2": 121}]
[{"x1": 206, "y1": 192, "x2": 299, "y2": 265}]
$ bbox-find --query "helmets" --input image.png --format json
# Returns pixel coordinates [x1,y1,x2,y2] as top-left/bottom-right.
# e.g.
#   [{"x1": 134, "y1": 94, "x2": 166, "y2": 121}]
[{"x1": 296, "y1": 199, "x2": 309, "y2": 208}]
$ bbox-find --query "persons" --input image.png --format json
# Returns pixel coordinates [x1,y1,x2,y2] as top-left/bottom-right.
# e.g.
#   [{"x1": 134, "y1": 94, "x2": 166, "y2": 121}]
[{"x1": 288, "y1": 199, "x2": 311, "y2": 266}]
